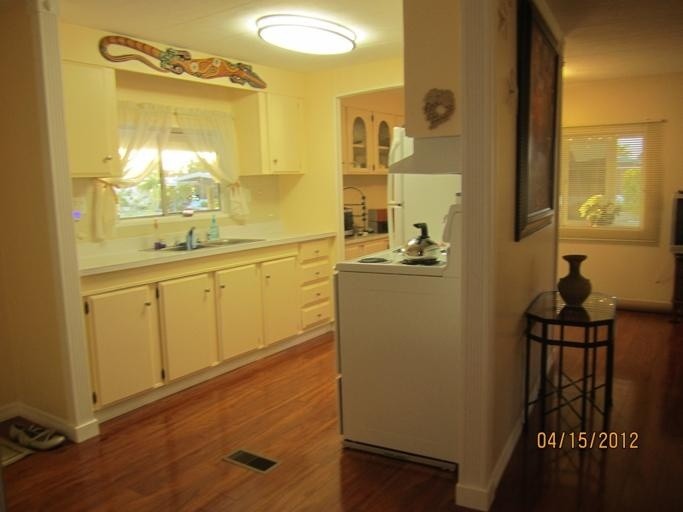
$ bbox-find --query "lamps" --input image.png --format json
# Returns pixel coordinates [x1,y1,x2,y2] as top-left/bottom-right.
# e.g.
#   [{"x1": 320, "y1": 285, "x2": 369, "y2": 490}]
[{"x1": 253, "y1": 14, "x2": 356, "y2": 56}]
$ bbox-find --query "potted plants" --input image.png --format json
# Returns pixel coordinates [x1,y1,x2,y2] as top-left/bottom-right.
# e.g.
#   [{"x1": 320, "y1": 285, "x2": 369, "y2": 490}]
[{"x1": 578, "y1": 195, "x2": 621, "y2": 227}]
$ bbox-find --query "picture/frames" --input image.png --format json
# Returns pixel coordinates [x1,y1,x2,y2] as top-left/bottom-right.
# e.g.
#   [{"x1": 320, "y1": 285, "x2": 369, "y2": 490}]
[{"x1": 514, "y1": 1, "x2": 561, "y2": 240}]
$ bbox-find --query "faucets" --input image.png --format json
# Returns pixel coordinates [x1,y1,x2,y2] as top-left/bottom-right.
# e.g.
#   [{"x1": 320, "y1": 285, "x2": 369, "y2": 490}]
[{"x1": 186, "y1": 227, "x2": 197, "y2": 246}]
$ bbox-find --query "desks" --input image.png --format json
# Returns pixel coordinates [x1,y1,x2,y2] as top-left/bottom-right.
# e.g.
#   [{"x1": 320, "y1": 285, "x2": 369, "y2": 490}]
[{"x1": 523, "y1": 289, "x2": 617, "y2": 458}]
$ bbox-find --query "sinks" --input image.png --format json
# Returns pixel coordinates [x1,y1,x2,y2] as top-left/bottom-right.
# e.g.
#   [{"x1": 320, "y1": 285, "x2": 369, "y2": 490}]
[
  {"x1": 163, "y1": 245, "x2": 208, "y2": 252},
  {"x1": 207, "y1": 239, "x2": 265, "y2": 246}
]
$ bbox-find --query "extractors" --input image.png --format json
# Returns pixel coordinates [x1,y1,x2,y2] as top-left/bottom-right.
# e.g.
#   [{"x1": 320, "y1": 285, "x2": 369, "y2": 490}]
[{"x1": 387, "y1": 131, "x2": 464, "y2": 175}]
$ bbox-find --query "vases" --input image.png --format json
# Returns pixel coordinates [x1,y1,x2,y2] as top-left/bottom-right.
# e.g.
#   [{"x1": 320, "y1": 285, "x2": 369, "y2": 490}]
[{"x1": 557, "y1": 254, "x2": 592, "y2": 307}]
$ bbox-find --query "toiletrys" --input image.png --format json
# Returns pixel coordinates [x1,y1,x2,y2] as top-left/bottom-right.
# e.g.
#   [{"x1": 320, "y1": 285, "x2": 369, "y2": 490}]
[{"x1": 208, "y1": 215, "x2": 219, "y2": 239}]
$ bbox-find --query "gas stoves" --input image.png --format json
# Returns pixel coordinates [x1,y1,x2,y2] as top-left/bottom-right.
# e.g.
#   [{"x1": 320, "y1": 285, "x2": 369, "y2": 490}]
[{"x1": 336, "y1": 241, "x2": 448, "y2": 277}]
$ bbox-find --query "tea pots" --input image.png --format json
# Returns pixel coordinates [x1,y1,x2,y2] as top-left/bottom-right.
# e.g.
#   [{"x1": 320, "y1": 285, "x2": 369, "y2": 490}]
[{"x1": 405, "y1": 223, "x2": 441, "y2": 261}]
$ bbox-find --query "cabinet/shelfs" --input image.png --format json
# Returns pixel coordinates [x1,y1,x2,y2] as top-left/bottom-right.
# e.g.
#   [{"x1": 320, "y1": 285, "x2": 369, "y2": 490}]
[
  {"x1": 158, "y1": 263, "x2": 259, "y2": 384},
  {"x1": 265, "y1": 92, "x2": 300, "y2": 174},
  {"x1": 260, "y1": 256, "x2": 299, "y2": 347},
  {"x1": 61, "y1": 60, "x2": 114, "y2": 176},
  {"x1": 86, "y1": 286, "x2": 154, "y2": 407},
  {"x1": 299, "y1": 239, "x2": 331, "y2": 330},
  {"x1": 345, "y1": 106, "x2": 393, "y2": 173}
]
